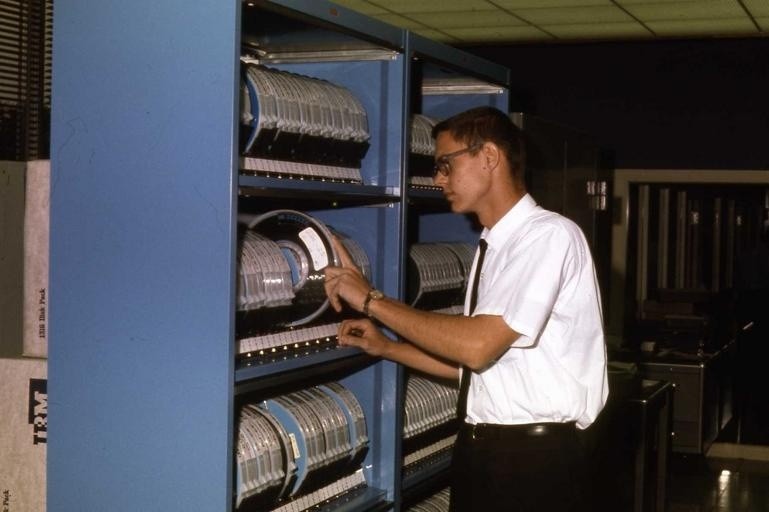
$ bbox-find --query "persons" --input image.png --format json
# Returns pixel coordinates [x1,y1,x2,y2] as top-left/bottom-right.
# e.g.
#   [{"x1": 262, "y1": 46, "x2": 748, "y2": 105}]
[{"x1": 320, "y1": 104, "x2": 611, "y2": 510}]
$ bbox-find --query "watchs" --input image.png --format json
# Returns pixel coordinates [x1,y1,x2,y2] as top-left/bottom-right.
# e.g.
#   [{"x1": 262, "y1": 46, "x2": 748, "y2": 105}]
[{"x1": 363, "y1": 287, "x2": 383, "y2": 318}]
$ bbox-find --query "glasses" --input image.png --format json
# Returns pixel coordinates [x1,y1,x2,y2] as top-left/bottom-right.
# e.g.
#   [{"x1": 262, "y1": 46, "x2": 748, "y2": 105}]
[{"x1": 432, "y1": 146, "x2": 476, "y2": 177}]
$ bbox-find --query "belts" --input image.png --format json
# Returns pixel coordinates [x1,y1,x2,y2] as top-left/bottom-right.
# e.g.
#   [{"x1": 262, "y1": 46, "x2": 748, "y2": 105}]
[{"x1": 472, "y1": 420, "x2": 577, "y2": 440}]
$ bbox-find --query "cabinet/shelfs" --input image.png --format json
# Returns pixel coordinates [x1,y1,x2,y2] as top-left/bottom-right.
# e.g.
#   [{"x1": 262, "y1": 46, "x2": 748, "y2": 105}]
[
  {"x1": 49, "y1": 2, "x2": 407, "y2": 511},
  {"x1": 400, "y1": 29, "x2": 514, "y2": 509}
]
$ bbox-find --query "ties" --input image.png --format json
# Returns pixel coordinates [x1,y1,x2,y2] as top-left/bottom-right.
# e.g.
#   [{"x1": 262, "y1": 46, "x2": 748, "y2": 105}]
[{"x1": 456, "y1": 238, "x2": 488, "y2": 422}]
